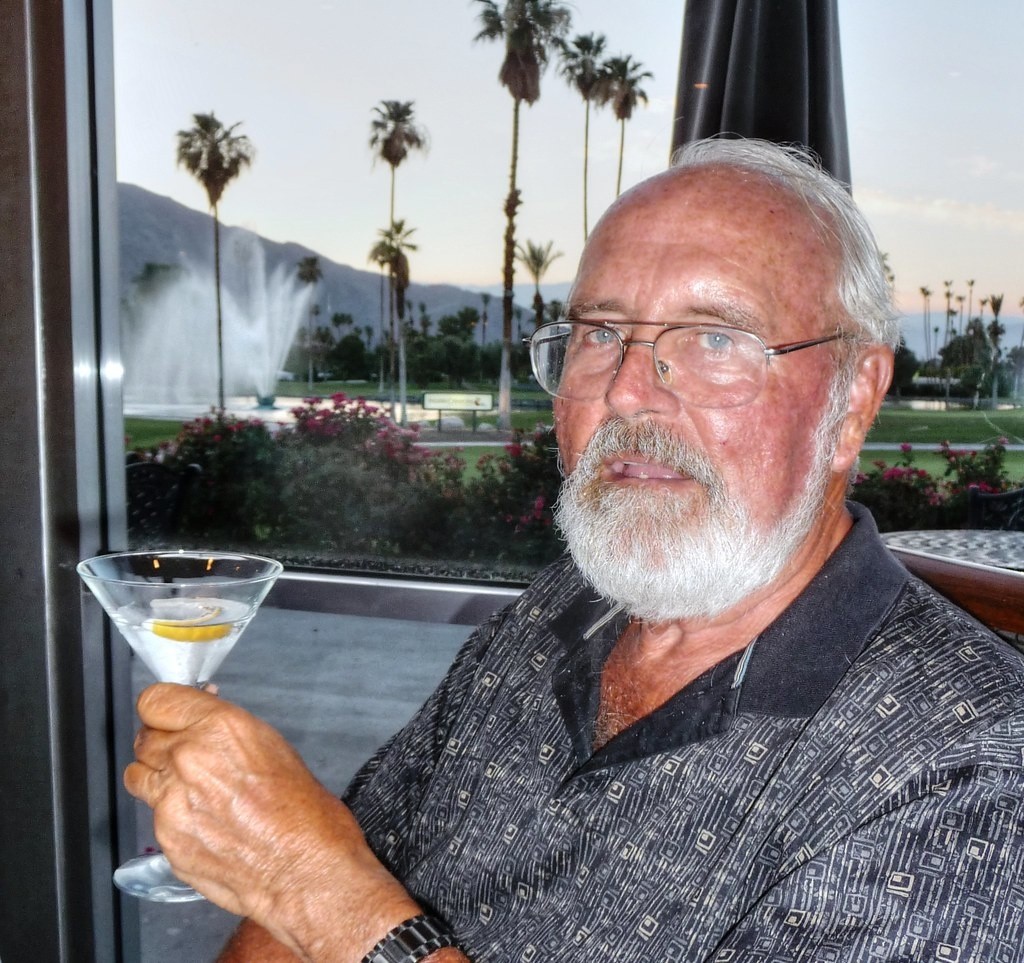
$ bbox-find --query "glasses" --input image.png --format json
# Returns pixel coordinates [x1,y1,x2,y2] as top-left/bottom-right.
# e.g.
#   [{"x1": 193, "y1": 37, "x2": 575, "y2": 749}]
[{"x1": 522, "y1": 321, "x2": 842, "y2": 407}]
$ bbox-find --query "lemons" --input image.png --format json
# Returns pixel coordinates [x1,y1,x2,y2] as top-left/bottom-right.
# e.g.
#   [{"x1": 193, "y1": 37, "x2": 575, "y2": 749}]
[{"x1": 151, "y1": 609, "x2": 232, "y2": 643}]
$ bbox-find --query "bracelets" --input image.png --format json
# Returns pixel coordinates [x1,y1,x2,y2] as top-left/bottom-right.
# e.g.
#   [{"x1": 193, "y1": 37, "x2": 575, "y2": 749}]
[{"x1": 360, "y1": 910, "x2": 462, "y2": 963}]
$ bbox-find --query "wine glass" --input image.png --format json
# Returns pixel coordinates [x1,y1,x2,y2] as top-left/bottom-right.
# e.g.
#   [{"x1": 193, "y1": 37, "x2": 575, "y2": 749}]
[{"x1": 76, "y1": 551, "x2": 286, "y2": 904}]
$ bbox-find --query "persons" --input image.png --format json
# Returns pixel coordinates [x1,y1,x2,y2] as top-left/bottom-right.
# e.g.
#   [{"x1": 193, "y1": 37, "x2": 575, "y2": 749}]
[{"x1": 121, "y1": 132, "x2": 1024, "y2": 963}]
